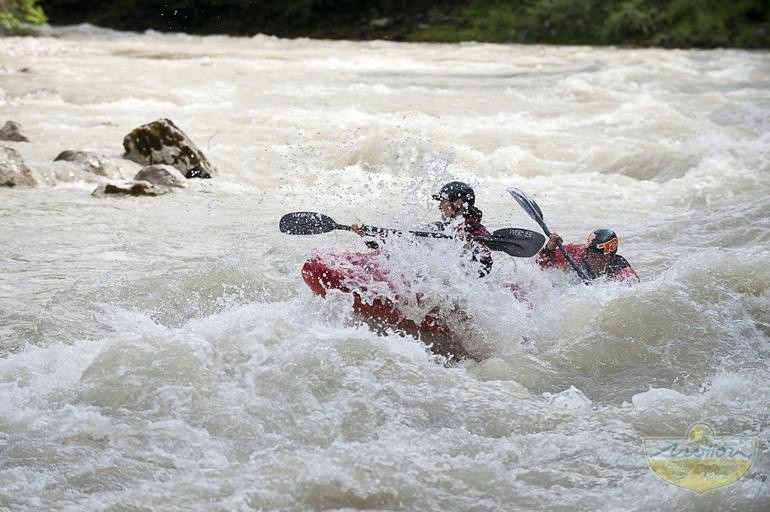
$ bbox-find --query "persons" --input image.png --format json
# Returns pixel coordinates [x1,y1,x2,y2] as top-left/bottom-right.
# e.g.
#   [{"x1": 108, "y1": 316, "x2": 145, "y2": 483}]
[
  {"x1": 534, "y1": 226, "x2": 641, "y2": 289},
  {"x1": 350, "y1": 176, "x2": 493, "y2": 280}
]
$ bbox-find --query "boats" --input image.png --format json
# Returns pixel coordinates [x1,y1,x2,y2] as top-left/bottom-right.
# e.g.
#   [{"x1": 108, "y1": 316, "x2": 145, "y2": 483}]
[{"x1": 299, "y1": 239, "x2": 643, "y2": 340}]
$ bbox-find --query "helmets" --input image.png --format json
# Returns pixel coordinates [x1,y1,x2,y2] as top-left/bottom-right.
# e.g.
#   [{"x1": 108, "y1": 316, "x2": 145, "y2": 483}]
[
  {"x1": 431, "y1": 181, "x2": 474, "y2": 209},
  {"x1": 588, "y1": 228, "x2": 618, "y2": 257}
]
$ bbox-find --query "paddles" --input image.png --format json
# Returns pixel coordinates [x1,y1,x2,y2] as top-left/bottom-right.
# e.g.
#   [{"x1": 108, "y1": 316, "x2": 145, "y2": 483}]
[
  {"x1": 505, "y1": 185, "x2": 593, "y2": 289},
  {"x1": 278, "y1": 211, "x2": 545, "y2": 257}
]
